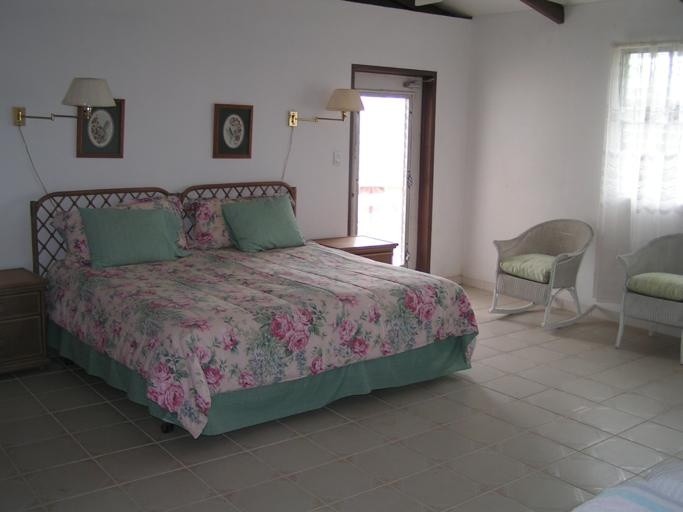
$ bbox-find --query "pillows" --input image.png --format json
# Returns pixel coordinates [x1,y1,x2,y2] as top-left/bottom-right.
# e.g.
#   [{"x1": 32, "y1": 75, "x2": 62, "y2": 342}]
[{"x1": 53, "y1": 194, "x2": 306, "y2": 270}]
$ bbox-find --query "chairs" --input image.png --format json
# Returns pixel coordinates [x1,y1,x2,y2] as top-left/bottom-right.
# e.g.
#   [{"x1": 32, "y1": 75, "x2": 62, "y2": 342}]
[{"x1": 487, "y1": 218, "x2": 682, "y2": 366}]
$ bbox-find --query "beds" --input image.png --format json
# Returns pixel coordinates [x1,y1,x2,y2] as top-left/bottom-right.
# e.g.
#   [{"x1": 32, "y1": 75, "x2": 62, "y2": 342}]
[{"x1": 30, "y1": 180, "x2": 480, "y2": 441}]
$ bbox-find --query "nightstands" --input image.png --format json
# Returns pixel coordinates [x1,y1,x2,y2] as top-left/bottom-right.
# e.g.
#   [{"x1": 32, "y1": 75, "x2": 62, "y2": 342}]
[
  {"x1": 0, "y1": 267, "x2": 51, "y2": 377},
  {"x1": 312, "y1": 236, "x2": 399, "y2": 265}
]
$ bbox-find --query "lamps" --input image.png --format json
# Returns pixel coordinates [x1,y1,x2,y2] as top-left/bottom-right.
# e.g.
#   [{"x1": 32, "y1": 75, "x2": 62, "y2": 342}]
[
  {"x1": 287, "y1": 88, "x2": 365, "y2": 127},
  {"x1": 12, "y1": 77, "x2": 117, "y2": 127}
]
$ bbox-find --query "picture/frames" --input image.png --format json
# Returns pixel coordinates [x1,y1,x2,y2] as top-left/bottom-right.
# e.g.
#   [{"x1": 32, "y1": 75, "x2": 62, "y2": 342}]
[
  {"x1": 211, "y1": 103, "x2": 254, "y2": 159},
  {"x1": 75, "y1": 98, "x2": 126, "y2": 159}
]
